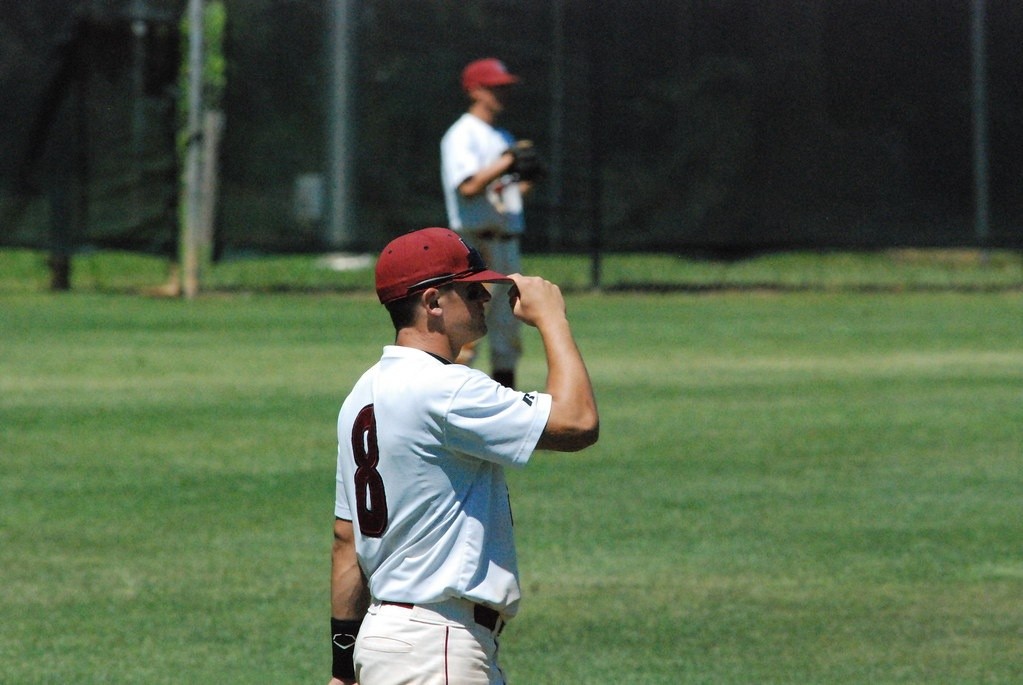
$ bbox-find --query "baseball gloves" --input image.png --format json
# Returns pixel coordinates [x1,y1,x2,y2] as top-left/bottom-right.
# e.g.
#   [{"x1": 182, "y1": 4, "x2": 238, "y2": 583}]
[{"x1": 500, "y1": 138, "x2": 542, "y2": 177}]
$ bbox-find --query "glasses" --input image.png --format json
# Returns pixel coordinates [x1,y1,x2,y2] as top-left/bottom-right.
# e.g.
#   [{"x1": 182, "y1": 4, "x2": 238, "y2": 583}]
[{"x1": 407, "y1": 248, "x2": 487, "y2": 290}]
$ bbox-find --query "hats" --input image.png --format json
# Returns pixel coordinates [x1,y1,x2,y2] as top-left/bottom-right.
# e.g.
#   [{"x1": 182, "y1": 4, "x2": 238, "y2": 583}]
[
  {"x1": 375, "y1": 227, "x2": 514, "y2": 303},
  {"x1": 461, "y1": 58, "x2": 517, "y2": 92}
]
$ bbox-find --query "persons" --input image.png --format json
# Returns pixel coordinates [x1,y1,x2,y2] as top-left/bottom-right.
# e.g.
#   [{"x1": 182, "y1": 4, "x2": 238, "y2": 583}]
[
  {"x1": 440, "y1": 58, "x2": 536, "y2": 390},
  {"x1": 328, "y1": 228, "x2": 600, "y2": 685}
]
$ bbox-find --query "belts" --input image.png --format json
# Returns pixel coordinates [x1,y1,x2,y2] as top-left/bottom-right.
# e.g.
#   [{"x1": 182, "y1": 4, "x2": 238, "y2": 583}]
[
  {"x1": 382, "y1": 599, "x2": 505, "y2": 637},
  {"x1": 471, "y1": 230, "x2": 519, "y2": 240}
]
